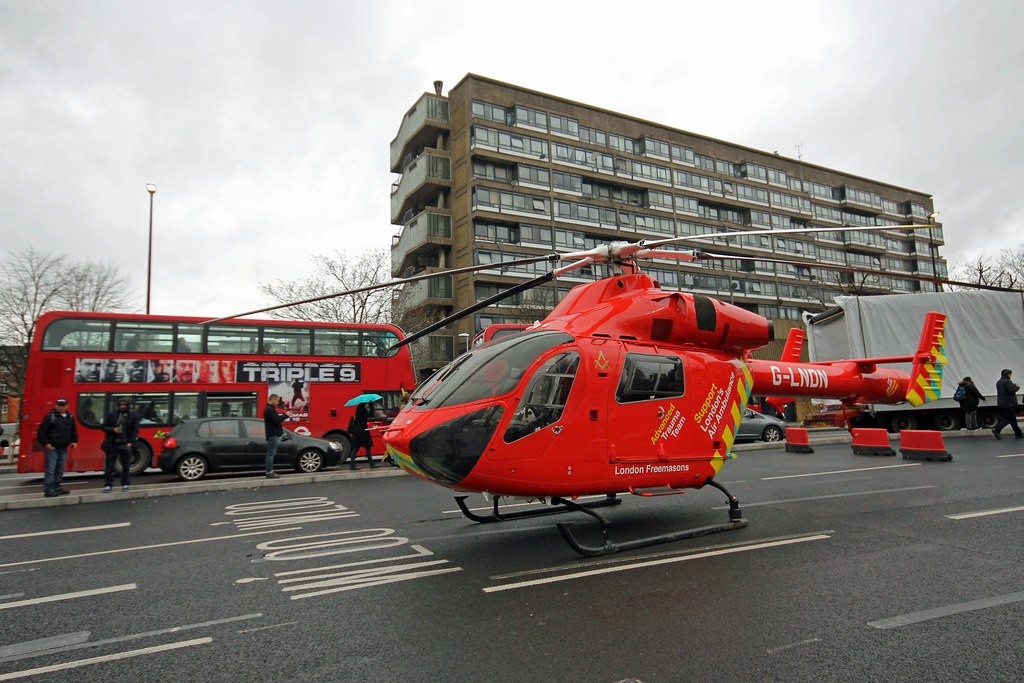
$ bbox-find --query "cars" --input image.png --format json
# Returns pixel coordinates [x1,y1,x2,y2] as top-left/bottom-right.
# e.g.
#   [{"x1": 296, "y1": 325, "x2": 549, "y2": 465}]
[
  {"x1": 156, "y1": 416, "x2": 344, "y2": 481},
  {"x1": 733, "y1": 407, "x2": 787, "y2": 443}
]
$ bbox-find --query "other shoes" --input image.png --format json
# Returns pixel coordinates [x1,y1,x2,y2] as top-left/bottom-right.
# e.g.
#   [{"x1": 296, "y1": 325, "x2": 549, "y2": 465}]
[
  {"x1": 992, "y1": 430, "x2": 1001, "y2": 439},
  {"x1": 350, "y1": 466, "x2": 362, "y2": 470},
  {"x1": 122, "y1": 484, "x2": 129, "y2": 492},
  {"x1": 56, "y1": 488, "x2": 70, "y2": 494},
  {"x1": 371, "y1": 463, "x2": 382, "y2": 468},
  {"x1": 266, "y1": 473, "x2": 280, "y2": 478},
  {"x1": 973, "y1": 426, "x2": 982, "y2": 430},
  {"x1": 1015, "y1": 435, "x2": 1024, "y2": 438},
  {"x1": 967, "y1": 428, "x2": 977, "y2": 432},
  {"x1": 104, "y1": 486, "x2": 111, "y2": 493},
  {"x1": 45, "y1": 491, "x2": 62, "y2": 497}
]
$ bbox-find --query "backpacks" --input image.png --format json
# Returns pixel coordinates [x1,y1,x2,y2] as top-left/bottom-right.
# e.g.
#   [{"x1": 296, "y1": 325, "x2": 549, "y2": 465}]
[{"x1": 954, "y1": 383, "x2": 970, "y2": 402}]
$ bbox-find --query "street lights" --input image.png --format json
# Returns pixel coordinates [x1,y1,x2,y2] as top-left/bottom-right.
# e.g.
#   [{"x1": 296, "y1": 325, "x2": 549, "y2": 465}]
[
  {"x1": 927, "y1": 211, "x2": 941, "y2": 292},
  {"x1": 144, "y1": 183, "x2": 158, "y2": 314}
]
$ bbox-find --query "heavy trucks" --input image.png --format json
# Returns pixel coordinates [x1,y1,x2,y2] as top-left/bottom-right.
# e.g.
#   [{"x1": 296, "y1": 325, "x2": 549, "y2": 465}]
[{"x1": 800, "y1": 287, "x2": 1024, "y2": 434}]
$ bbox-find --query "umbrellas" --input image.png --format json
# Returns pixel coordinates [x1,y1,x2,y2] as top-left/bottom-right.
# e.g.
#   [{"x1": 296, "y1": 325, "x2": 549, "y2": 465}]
[{"x1": 344, "y1": 394, "x2": 383, "y2": 407}]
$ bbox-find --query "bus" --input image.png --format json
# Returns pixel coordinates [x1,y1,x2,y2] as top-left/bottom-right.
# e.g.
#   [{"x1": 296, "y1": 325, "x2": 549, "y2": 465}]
[{"x1": 14, "y1": 309, "x2": 423, "y2": 477}]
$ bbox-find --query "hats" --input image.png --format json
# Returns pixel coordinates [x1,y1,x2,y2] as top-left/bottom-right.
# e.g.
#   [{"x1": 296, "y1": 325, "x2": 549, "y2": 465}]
[{"x1": 57, "y1": 398, "x2": 68, "y2": 406}]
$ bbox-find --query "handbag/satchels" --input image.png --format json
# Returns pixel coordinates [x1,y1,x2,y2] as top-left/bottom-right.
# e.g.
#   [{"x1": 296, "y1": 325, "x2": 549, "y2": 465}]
[{"x1": 348, "y1": 419, "x2": 365, "y2": 434}]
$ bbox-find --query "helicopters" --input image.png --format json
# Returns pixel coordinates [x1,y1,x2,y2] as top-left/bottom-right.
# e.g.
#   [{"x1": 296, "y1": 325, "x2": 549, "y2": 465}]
[{"x1": 194, "y1": 221, "x2": 1023, "y2": 560}]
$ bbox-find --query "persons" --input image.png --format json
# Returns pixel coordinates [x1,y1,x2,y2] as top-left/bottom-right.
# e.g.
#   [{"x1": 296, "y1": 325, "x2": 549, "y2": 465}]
[
  {"x1": 125, "y1": 334, "x2": 272, "y2": 355},
  {"x1": 957, "y1": 376, "x2": 987, "y2": 431},
  {"x1": 80, "y1": 398, "x2": 237, "y2": 424},
  {"x1": 350, "y1": 400, "x2": 382, "y2": 470},
  {"x1": 75, "y1": 358, "x2": 236, "y2": 384},
  {"x1": 400, "y1": 393, "x2": 410, "y2": 410},
  {"x1": 991, "y1": 369, "x2": 1024, "y2": 440},
  {"x1": 97, "y1": 397, "x2": 140, "y2": 493},
  {"x1": 263, "y1": 394, "x2": 292, "y2": 479},
  {"x1": 37, "y1": 397, "x2": 79, "y2": 497}
]
